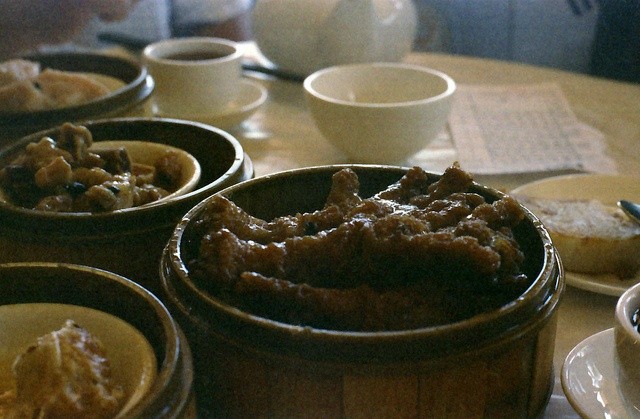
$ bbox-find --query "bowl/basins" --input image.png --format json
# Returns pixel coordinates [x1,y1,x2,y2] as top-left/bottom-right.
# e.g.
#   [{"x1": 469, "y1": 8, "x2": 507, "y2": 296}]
[
  {"x1": 0, "y1": 141, "x2": 201, "y2": 218},
  {"x1": 303, "y1": 61, "x2": 455, "y2": 165},
  {"x1": 0, "y1": 303, "x2": 156, "y2": 419}
]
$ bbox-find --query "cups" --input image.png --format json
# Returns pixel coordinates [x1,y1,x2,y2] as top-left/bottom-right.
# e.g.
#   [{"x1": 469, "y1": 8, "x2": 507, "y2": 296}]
[
  {"x1": 615, "y1": 282, "x2": 640, "y2": 385},
  {"x1": 143, "y1": 37, "x2": 242, "y2": 115}
]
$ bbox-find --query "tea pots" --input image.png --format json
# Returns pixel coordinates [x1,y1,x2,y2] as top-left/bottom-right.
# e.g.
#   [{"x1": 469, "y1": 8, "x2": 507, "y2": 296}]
[{"x1": 253, "y1": 1, "x2": 419, "y2": 79}]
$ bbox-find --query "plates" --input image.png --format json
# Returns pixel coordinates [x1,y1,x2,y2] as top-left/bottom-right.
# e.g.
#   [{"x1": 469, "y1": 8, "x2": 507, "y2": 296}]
[
  {"x1": 507, "y1": 173, "x2": 640, "y2": 296},
  {"x1": 154, "y1": 79, "x2": 268, "y2": 128},
  {"x1": 560, "y1": 327, "x2": 635, "y2": 419}
]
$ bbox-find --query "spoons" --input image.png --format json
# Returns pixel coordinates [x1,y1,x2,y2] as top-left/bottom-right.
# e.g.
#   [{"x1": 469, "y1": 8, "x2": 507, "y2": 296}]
[{"x1": 618, "y1": 199, "x2": 639, "y2": 225}]
